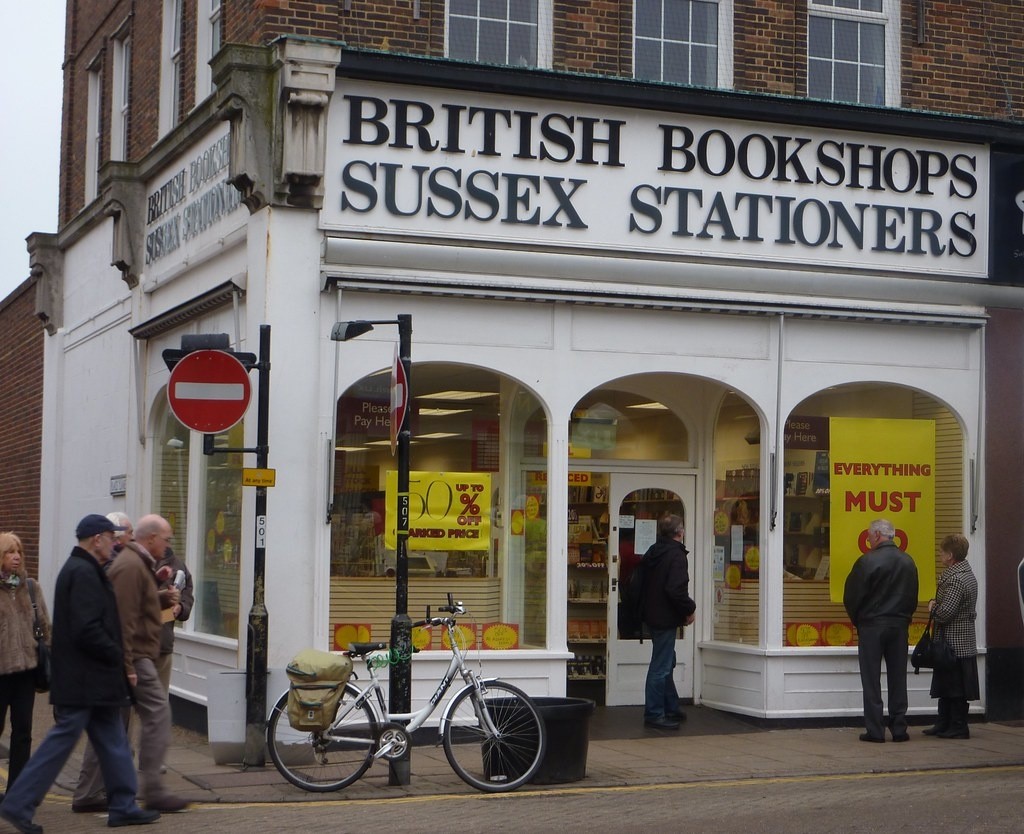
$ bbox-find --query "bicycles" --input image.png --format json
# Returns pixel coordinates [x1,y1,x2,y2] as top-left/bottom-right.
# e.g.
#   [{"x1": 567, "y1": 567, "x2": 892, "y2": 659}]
[{"x1": 267, "y1": 592, "x2": 547, "y2": 793}]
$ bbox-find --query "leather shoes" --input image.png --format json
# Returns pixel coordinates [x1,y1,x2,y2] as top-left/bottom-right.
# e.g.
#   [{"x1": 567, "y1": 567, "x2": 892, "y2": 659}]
[
  {"x1": 644, "y1": 713, "x2": 679, "y2": 730},
  {"x1": 665, "y1": 710, "x2": 687, "y2": 724},
  {"x1": 108, "y1": 804, "x2": 161, "y2": 829},
  {"x1": 1, "y1": 804, "x2": 44, "y2": 834}
]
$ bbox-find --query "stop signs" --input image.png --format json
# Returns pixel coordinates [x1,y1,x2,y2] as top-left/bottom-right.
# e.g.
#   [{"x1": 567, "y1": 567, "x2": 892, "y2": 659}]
[
  {"x1": 388, "y1": 341, "x2": 408, "y2": 456},
  {"x1": 167, "y1": 349, "x2": 251, "y2": 434}
]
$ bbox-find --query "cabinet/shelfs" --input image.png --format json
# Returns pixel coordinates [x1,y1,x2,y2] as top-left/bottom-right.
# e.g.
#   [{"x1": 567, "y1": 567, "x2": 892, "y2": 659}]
[{"x1": 566, "y1": 493, "x2": 829, "y2": 683}]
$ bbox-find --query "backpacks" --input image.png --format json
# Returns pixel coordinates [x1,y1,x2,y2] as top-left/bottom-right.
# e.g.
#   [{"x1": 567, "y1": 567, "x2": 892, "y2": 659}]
[{"x1": 620, "y1": 548, "x2": 672, "y2": 623}]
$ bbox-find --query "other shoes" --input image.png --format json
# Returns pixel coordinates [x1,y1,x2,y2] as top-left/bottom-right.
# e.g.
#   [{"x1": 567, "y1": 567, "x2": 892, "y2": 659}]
[
  {"x1": 71, "y1": 795, "x2": 110, "y2": 813},
  {"x1": 859, "y1": 731, "x2": 886, "y2": 745},
  {"x1": 144, "y1": 794, "x2": 187, "y2": 812},
  {"x1": 894, "y1": 733, "x2": 909, "y2": 744}
]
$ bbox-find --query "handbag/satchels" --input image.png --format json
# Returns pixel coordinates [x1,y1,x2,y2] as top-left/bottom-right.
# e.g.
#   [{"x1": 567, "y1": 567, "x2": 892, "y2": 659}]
[
  {"x1": 26, "y1": 578, "x2": 50, "y2": 692},
  {"x1": 911, "y1": 607, "x2": 940, "y2": 668},
  {"x1": 286, "y1": 650, "x2": 352, "y2": 733},
  {"x1": 933, "y1": 608, "x2": 956, "y2": 677}
]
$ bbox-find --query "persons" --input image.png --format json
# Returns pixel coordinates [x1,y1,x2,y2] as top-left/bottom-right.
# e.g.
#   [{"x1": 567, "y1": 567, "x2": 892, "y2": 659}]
[
  {"x1": 641, "y1": 516, "x2": 698, "y2": 730},
  {"x1": 920, "y1": 533, "x2": 980, "y2": 739},
  {"x1": 843, "y1": 518, "x2": 919, "y2": 742},
  {"x1": 0, "y1": 530, "x2": 52, "y2": 803},
  {"x1": 0, "y1": 515, "x2": 161, "y2": 834},
  {"x1": 73, "y1": 513, "x2": 201, "y2": 814}
]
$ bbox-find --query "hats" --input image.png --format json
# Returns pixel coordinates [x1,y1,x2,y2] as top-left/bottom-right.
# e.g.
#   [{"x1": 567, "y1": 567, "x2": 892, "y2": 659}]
[{"x1": 75, "y1": 514, "x2": 130, "y2": 542}]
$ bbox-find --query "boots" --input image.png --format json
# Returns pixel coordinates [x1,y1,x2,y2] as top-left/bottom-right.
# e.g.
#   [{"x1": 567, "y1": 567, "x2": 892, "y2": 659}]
[
  {"x1": 922, "y1": 691, "x2": 957, "y2": 735},
  {"x1": 938, "y1": 702, "x2": 970, "y2": 740}
]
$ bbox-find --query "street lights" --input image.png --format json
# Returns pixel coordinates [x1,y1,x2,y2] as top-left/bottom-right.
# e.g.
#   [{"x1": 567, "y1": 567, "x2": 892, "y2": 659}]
[
  {"x1": 162, "y1": 324, "x2": 271, "y2": 770},
  {"x1": 330, "y1": 315, "x2": 413, "y2": 786}
]
